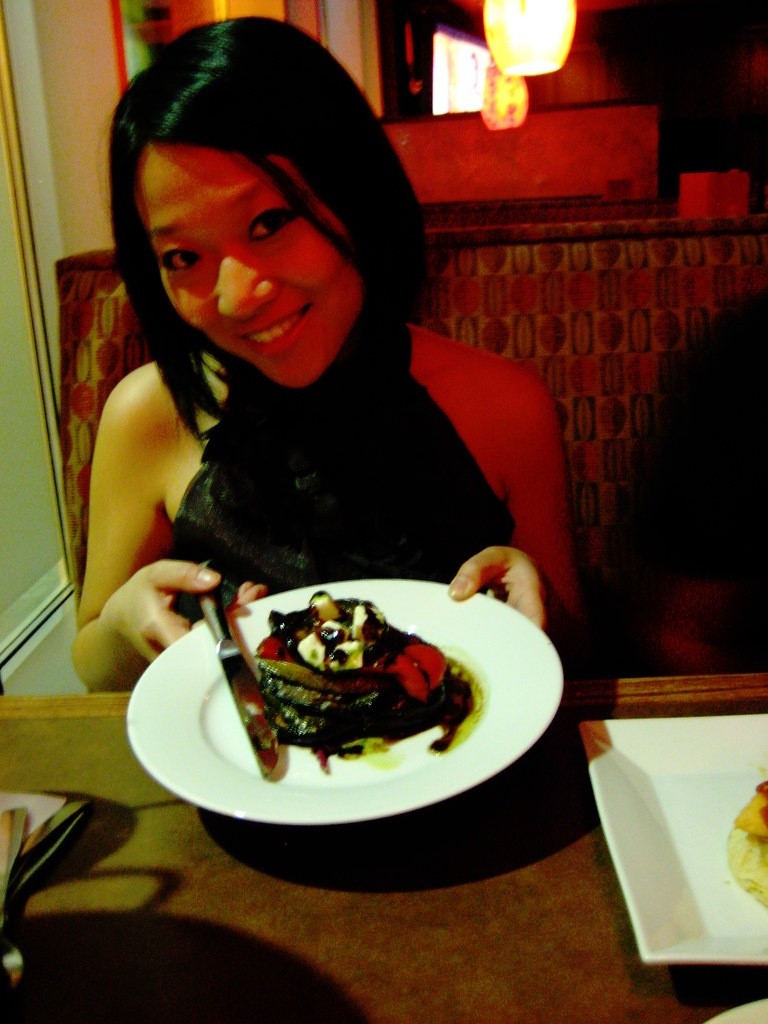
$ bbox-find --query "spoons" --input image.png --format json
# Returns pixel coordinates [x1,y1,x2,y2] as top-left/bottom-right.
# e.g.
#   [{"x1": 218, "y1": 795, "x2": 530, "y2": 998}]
[{"x1": 0, "y1": 807, "x2": 27, "y2": 992}]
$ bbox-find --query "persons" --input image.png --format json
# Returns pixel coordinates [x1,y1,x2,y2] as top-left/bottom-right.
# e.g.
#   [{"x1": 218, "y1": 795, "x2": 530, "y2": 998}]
[
  {"x1": 69, "y1": 17, "x2": 580, "y2": 696},
  {"x1": 620, "y1": 283, "x2": 767, "y2": 672}
]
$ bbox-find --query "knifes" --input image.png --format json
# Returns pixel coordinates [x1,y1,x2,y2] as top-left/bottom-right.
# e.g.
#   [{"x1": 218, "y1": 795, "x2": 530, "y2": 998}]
[{"x1": 195, "y1": 559, "x2": 278, "y2": 777}]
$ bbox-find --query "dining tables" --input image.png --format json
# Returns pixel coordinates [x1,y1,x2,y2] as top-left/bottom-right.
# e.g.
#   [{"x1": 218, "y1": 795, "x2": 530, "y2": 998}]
[{"x1": 0, "y1": 671, "x2": 768, "y2": 1024}]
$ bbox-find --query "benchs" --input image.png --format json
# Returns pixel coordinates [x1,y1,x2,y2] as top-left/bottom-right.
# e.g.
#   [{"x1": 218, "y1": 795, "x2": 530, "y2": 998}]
[{"x1": 53, "y1": 213, "x2": 768, "y2": 682}]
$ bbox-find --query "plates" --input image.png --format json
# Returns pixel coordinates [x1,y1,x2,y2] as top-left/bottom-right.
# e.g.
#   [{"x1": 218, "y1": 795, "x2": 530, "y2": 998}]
[
  {"x1": 578, "y1": 713, "x2": 768, "y2": 967},
  {"x1": 127, "y1": 577, "x2": 563, "y2": 825}
]
